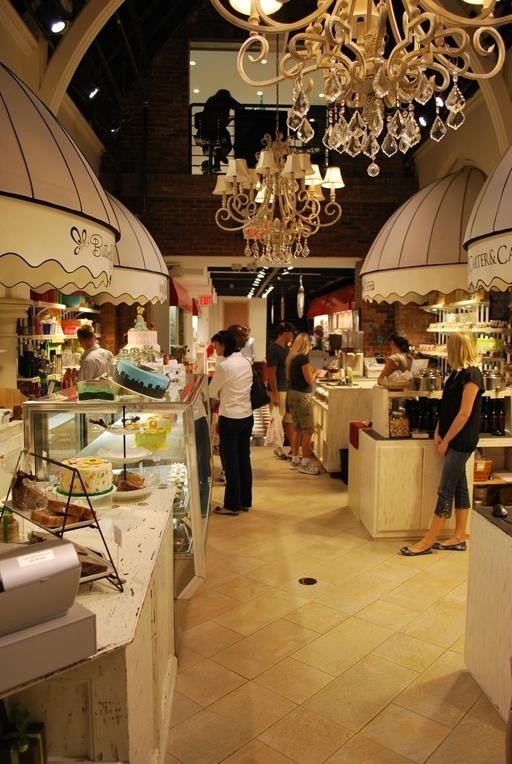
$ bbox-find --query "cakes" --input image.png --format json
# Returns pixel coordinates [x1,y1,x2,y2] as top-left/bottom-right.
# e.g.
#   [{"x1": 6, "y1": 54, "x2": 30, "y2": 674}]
[
  {"x1": 113, "y1": 470, "x2": 145, "y2": 490},
  {"x1": 58, "y1": 456, "x2": 112, "y2": 496},
  {"x1": 78, "y1": 303, "x2": 186, "y2": 452}
]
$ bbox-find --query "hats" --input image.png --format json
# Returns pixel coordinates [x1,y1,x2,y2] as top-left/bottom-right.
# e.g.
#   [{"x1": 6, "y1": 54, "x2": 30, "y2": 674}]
[{"x1": 277, "y1": 320, "x2": 299, "y2": 334}]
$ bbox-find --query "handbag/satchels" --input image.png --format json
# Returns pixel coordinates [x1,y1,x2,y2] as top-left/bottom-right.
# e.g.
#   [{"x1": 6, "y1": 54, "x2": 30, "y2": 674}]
[{"x1": 251, "y1": 365, "x2": 271, "y2": 410}]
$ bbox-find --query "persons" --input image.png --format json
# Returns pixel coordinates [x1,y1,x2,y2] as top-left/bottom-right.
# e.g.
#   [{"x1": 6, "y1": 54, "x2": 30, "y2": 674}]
[
  {"x1": 210, "y1": 322, "x2": 327, "y2": 475},
  {"x1": 76, "y1": 324, "x2": 114, "y2": 382},
  {"x1": 400, "y1": 330, "x2": 486, "y2": 556},
  {"x1": 207, "y1": 330, "x2": 254, "y2": 515},
  {"x1": 378, "y1": 336, "x2": 412, "y2": 408}
]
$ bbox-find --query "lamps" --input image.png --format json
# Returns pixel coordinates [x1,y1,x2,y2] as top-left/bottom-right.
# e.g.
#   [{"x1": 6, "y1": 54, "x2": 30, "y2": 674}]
[
  {"x1": 213, "y1": 0, "x2": 512, "y2": 173},
  {"x1": 209, "y1": 35, "x2": 346, "y2": 271}
]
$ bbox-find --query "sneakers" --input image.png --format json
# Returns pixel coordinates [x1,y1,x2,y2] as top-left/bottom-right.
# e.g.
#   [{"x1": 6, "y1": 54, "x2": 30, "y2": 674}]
[{"x1": 271, "y1": 447, "x2": 320, "y2": 475}]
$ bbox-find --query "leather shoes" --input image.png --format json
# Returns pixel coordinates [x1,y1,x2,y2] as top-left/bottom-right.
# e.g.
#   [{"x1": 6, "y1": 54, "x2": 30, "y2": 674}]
[
  {"x1": 400, "y1": 547, "x2": 433, "y2": 556},
  {"x1": 213, "y1": 504, "x2": 249, "y2": 515},
  {"x1": 432, "y1": 542, "x2": 466, "y2": 551}
]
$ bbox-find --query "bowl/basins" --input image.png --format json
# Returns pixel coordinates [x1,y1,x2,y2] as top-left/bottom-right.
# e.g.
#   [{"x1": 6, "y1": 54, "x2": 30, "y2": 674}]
[
  {"x1": 112, "y1": 471, "x2": 162, "y2": 500},
  {"x1": 62, "y1": 294, "x2": 81, "y2": 308},
  {"x1": 97, "y1": 447, "x2": 152, "y2": 464}
]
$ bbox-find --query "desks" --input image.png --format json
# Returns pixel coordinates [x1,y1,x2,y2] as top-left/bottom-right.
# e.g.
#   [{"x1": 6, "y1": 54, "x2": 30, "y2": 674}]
[
  {"x1": 465, "y1": 507, "x2": 512, "y2": 727},
  {"x1": 0, "y1": 485, "x2": 178, "y2": 764}
]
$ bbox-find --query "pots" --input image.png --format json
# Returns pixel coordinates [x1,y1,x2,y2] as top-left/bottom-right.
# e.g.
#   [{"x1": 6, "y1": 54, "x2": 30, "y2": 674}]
[
  {"x1": 481, "y1": 369, "x2": 503, "y2": 390},
  {"x1": 416, "y1": 367, "x2": 442, "y2": 391}
]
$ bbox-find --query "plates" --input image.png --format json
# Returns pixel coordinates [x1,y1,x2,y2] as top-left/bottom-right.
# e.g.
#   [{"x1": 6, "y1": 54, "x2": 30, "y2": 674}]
[
  {"x1": 52, "y1": 534, "x2": 113, "y2": 585},
  {"x1": 106, "y1": 422, "x2": 146, "y2": 435},
  {"x1": 1, "y1": 496, "x2": 101, "y2": 532}
]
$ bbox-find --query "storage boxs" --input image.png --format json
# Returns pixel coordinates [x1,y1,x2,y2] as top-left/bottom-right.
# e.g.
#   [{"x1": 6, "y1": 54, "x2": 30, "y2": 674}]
[{"x1": 496, "y1": 487, "x2": 512, "y2": 506}]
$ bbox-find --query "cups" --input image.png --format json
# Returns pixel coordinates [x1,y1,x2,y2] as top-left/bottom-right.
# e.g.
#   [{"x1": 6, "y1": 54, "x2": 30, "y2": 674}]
[
  {"x1": 345, "y1": 352, "x2": 364, "y2": 377},
  {"x1": 392, "y1": 398, "x2": 399, "y2": 411}
]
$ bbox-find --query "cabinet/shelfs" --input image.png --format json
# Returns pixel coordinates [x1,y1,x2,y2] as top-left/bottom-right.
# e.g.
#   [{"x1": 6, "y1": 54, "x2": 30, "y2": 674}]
[
  {"x1": 17, "y1": 299, "x2": 102, "y2": 398},
  {"x1": 476, "y1": 391, "x2": 512, "y2": 520},
  {"x1": 310, "y1": 385, "x2": 372, "y2": 473},
  {"x1": 346, "y1": 386, "x2": 476, "y2": 542},
  {"x1": 22, "y1": 373, "x2": 214, "y2": 601},
  {"x1": 419, "y1": 299, "x2": 511, "y2": 393},
  {"x1": 0, "y1": 419, "x2": 25, "y2": 500}
]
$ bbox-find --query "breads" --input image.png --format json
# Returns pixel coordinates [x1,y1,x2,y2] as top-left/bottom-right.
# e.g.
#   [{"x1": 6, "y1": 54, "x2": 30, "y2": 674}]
[{"x1": 30, "y1": 498, "x2": 107, "y2": 575}]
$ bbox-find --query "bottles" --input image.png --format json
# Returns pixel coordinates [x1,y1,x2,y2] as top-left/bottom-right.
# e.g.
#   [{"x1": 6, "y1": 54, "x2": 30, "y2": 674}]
[
  {"x1": 15, "y1": 315, "x2": 84, "y2": 399},
  {"x1": 328, "y1": 365, "x2": 354, "y2": 386},
  {"x1": 0, "y1": 514, "x2": 20, "y2": 542},
  {"x1": 429, "y1": 319, "x2": 508, "y2": 330},
  {"x1": 322, "y1": 357, "x2": 327, "y2": 370},
  {"x1": 389, "y1": 411, "x2": 410, "y2": 439},
  {"x1": 172, "y1": 506, "x2": 193, "y2": 553},
  {"x1": 405, "y1": 397, "x2": 507, "y2": 436}
]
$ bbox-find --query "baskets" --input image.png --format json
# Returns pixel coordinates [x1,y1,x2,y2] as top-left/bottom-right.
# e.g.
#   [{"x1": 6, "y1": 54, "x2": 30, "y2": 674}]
[
  {"x1": 378, "y1": 379, "x2": 412, "y2": 389},
  {"x1": 474, "y1": 456, "x2": 493, "y2": 481}
]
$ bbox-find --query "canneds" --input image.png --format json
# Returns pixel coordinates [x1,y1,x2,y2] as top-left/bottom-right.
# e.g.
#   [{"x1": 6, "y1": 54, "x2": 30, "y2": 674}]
[{"x1": 328, "y1": 368, "x2": 339, "y2": 378}]
